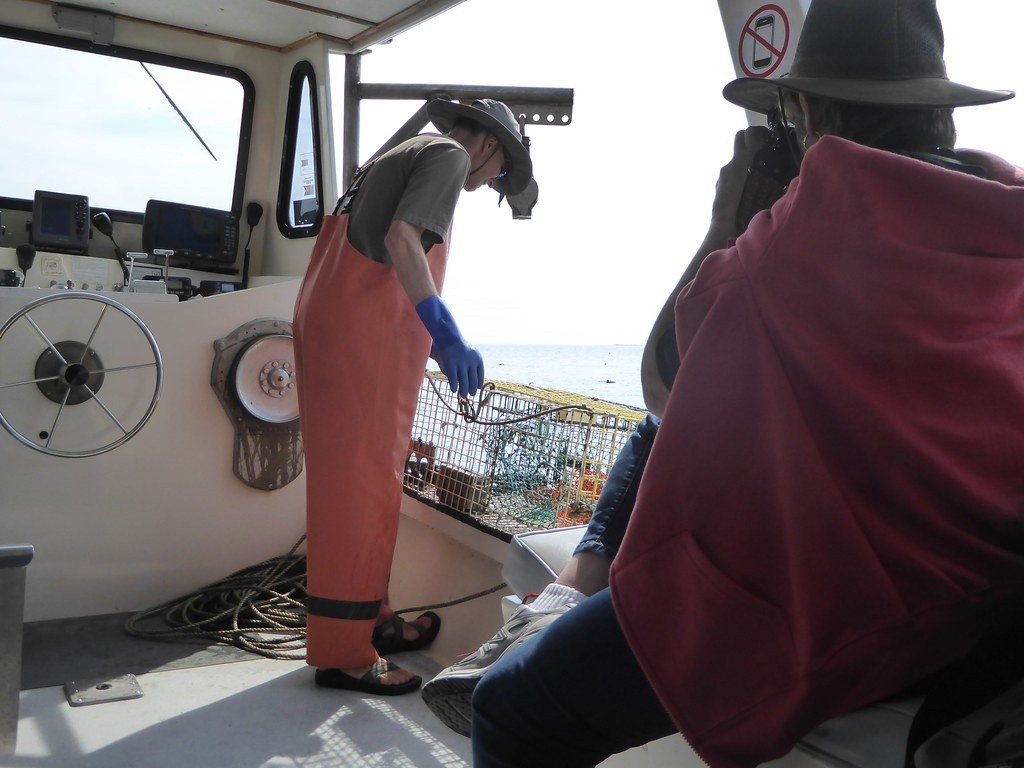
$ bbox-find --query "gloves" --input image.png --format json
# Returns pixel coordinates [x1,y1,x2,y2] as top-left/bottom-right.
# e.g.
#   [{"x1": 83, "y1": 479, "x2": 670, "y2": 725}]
[{"x1": 416, "y1": 295, "x2": 485, "y2": 399}]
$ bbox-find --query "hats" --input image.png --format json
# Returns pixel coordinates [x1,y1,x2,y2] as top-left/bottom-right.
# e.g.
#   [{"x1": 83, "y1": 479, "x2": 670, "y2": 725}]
[
  {"x1": 722, "y1": 0, "x2": 1016, "y2": 116},
  {"x1": 426, "y1": 98, "x2": 533, "y2": 196}
]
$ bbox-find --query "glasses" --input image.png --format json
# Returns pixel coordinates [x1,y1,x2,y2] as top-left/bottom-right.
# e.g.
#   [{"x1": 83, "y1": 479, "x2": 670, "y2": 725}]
[{"x1": 495, "y1": 142, "x2": 508, "y2": 181}]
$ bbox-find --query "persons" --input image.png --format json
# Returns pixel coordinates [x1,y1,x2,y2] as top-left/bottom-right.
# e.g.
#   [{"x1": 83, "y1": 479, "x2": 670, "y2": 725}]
[
  {"x1": 291, "y1": 99, "x2": 533, "y2": 695},
  {"x1": 420, "y1": 0, "x2": 1024, "y2": 768}
]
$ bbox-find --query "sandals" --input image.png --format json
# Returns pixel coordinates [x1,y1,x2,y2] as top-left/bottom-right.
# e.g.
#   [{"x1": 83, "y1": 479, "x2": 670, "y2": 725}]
[
  {"x1": 372, "y1": 611, "x2": 442, "y2": 656},
  {"x1": 315, "y1": 659, "x2": 421, "y2": 696}
]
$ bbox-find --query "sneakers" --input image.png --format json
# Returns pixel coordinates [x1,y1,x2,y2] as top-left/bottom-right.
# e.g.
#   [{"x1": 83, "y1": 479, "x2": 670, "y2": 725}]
[{"x1": 420, "y1": 592, "x2": 578, "y2": 739}]
